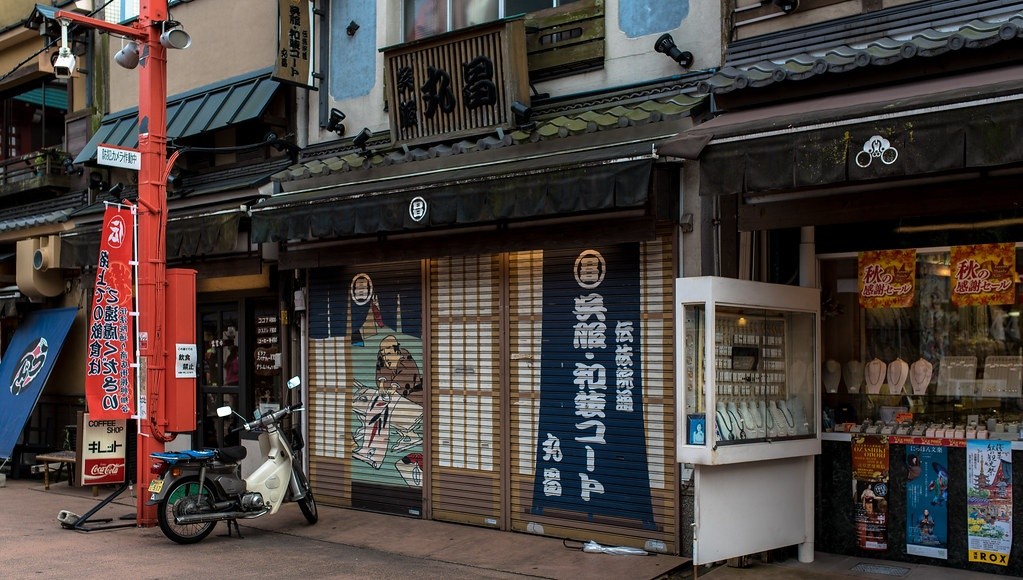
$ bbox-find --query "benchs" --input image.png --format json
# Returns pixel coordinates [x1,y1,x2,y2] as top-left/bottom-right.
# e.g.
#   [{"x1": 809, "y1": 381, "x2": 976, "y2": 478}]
[{"x1": 35, "y1": 451, "x2": 77, "y2": 490}]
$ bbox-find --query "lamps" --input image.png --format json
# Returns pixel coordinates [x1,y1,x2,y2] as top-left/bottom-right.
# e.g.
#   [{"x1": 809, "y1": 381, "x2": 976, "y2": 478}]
[
  {"x1": 353, "y1": 127, "x2": 374, "y2": 156},
  {"x1": 509, "y1": 101, "x2": 536, "y2": 130},
  {"x1": 326, "y1": 108, "x2": 347, "y2": 136},
  {"x1": 771, "y1": 0, "x2": 799, "y2": 14},
  {"x1": 654, "y1": 33, "x2": 695, "y2": 71},
  {"x1": 345, "y1": 21, "x2": 360, "y2": 36},
  {"x1": 114, "y1": 34, "x2": 141, "y2": 71},
  {"x1": 159, "y1": 20, "x2": 191, "y2": 50}
]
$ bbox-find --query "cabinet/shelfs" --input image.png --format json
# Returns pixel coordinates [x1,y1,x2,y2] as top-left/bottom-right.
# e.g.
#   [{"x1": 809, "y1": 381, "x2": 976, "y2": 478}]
[{"x1": 670, "y1": 273, "x2": 823, "y2": 580}]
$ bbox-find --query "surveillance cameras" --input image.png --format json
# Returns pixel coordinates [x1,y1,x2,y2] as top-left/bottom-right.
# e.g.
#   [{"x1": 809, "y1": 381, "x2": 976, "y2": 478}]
[{"x1": 54, "y1": 53, "x2": 76, "y2": 79}]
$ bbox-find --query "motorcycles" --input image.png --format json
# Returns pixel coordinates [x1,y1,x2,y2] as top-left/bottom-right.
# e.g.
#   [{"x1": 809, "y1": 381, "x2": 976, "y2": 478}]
[{"x1": 145, "y1": 375, "x2": 318, "y2": 545}]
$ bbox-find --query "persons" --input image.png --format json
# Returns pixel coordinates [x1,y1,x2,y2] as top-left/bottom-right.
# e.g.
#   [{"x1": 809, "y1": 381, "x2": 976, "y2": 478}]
[
  {"x1": 205, "y1": 341, "x2": 216, "y2": 411},
  {"x1": 919, "y1": 509, "x2": 934, "y2": 536},
  {"x1": 858, "y1": 484, "x2": 883, "y2": 513},
  {"x1": 83, "y1": 205, "x2": 135, "y2": 422},
  {"x1": 223, "y1": 344, "x2": 239, "y2": 419}
]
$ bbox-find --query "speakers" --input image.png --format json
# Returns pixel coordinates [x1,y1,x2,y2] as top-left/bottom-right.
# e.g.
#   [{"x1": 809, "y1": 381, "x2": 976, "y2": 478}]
[
  {"x1": 113, "y1": 42, "x2": 140, "y2": 69},
  {"x1": 159, "y1": 30, "x2": 193, "y2": 50}
]
